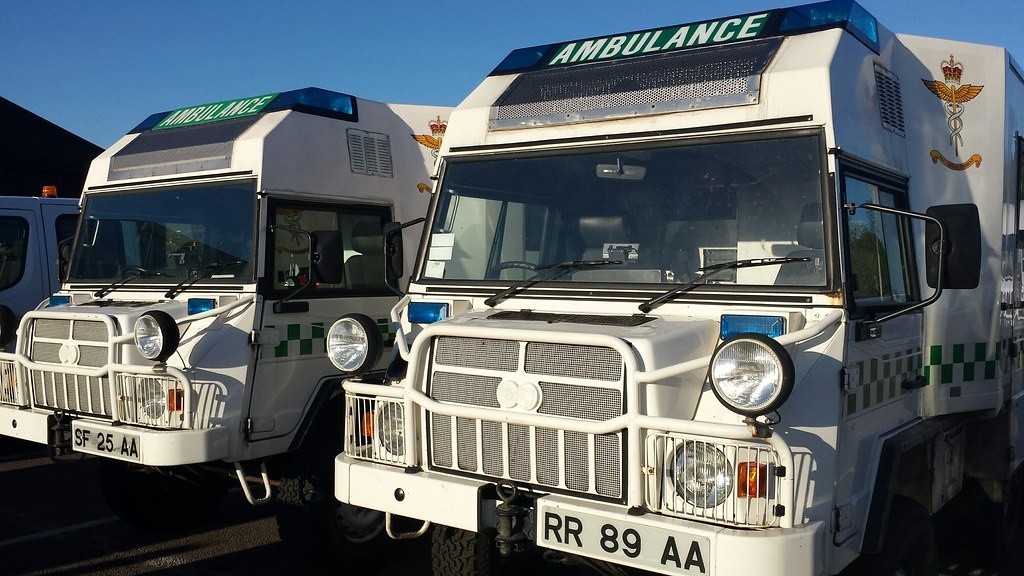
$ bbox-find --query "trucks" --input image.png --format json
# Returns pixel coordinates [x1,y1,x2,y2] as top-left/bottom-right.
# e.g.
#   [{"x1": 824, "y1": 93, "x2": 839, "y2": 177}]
[
  {"x1": 335, "y1": 0, "x2": 1024, "y2": 576},
  {"x1": 1, "y1": 88, "x2": 524, "y2": 576}
]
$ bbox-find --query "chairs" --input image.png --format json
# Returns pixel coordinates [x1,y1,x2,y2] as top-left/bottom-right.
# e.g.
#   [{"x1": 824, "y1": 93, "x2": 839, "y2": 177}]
[
  {"x1": 578, "y1": 209, "x2": 673, "y2": 277},
  {"x1": 773, "y1": 202, "x2": 875, "y2": 298},
  {"x1": 213, "y1": 243, "x2": 247, "y2": 277},
  {"x1": 0, "y1": 239, "x2": 23, "y2": 288},
  {"x1": 332, "y1": 221, "x2": 385, "y2": 288}
]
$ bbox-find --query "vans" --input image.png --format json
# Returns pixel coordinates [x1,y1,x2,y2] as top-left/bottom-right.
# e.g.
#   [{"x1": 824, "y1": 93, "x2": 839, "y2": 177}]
[{"x1": 0, "y1": 185, "x2": 139, "y2": 351}]
